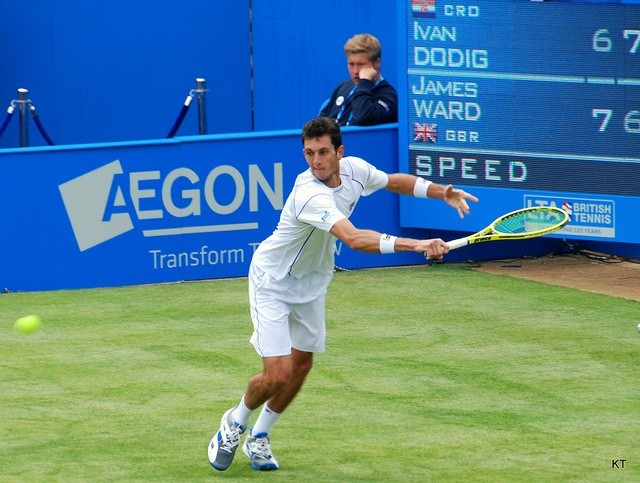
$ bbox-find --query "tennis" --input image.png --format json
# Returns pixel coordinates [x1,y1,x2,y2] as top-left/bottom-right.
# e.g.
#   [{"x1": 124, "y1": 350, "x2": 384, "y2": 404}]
[{"x1": 15, "y1": 316, "x2": 41, "y2": 334}]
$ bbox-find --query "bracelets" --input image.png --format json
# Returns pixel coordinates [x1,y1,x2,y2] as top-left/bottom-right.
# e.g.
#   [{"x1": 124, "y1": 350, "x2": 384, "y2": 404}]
[
  {"x1": 413, "y1": 176, "x2": 432, "y2": 199},
  {"x1": 379, "y1": 233, "x2": 397, "y2": 254}
]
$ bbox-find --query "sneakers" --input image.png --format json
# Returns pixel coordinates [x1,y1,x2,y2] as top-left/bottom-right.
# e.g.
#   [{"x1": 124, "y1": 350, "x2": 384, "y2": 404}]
[
  {"x1": 243, "y1": 428, "x2": 279, "y2": 471},
  {"x1": 207, "y1": 404, "x2": 247, "y2": 470}
]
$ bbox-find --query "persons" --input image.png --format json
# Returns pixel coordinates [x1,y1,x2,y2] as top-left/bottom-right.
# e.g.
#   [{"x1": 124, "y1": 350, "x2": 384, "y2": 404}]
[
  {"x1": 206, "y1": 117, "x2": 479, "y2": 472},
  {"x1": 319, "y1": 33, "x2": 398, "y2": 127}
]
